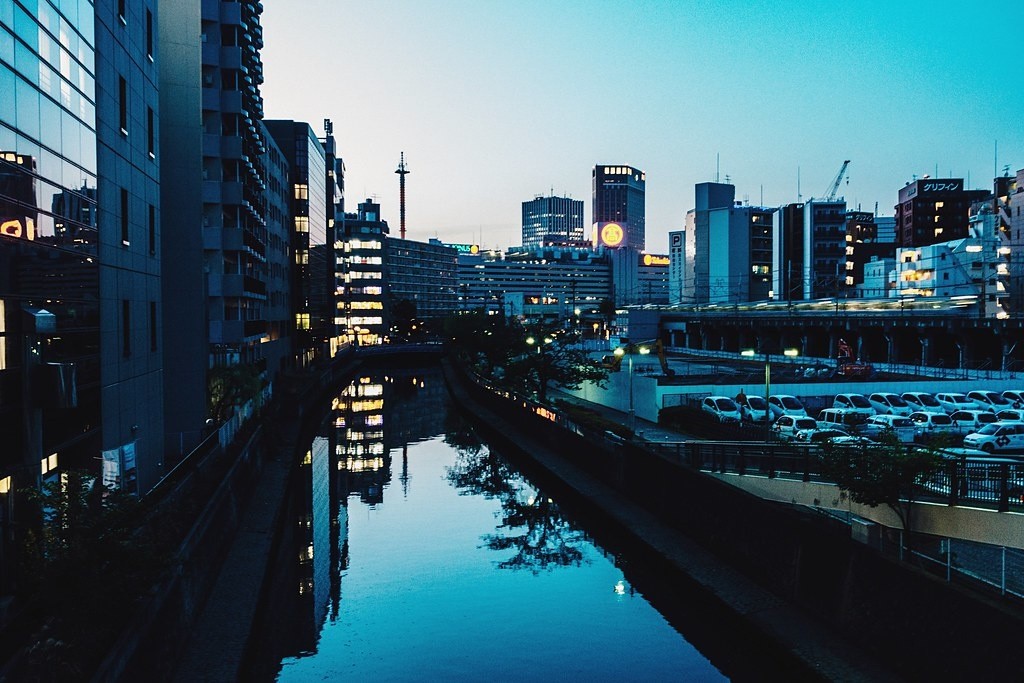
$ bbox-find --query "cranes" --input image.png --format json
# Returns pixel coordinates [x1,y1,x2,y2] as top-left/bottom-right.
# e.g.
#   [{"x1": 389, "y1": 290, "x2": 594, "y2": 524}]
[{"x1": 818, "y1": 156, "x2": 852, "y2": 203}]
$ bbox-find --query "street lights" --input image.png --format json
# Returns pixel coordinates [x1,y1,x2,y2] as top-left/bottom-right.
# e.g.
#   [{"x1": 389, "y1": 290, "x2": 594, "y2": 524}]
[
  {"x1": 739, "y1": 348, "x2": 800, "y2": 443},
  {"x1": 614, "y1": 338, "x2": 650, "y2": 431}
]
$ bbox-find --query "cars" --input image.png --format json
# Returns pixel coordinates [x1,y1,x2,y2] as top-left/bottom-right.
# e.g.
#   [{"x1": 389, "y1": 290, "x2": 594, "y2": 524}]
[
  {"x1": 824, "y1": 435, "x2": 873, "y2": 456},
  {"x1": 1002, "y1": 390, "x2": 1024, "y2": 406},
  {"x1": 766, "y1": 394, "x2": 808, "y2": 418},
  {"x1": 772, "y1": 415, "x2": 819, "y2": 443},
  {"x1": 994, "y1": 409, "x2": 1024, "y2": 422},
  {"x1": 734, "y1": 394, "x2": 775, "y2": 423},
  {"x1": 868, "y1": 393, "x2": 914, "y2": 417},
  {"x1": 900, "y1": 390, "x2": 946, "y2": 415},
  {"x1": 935, "y1": 392, "x2": 980, "y2": 413},
  {"x1": 948, "y1": 409, "x2": 999, "y2": 438},
  {"x1": 701, "y1": 396, "x2": 742, "y2": 425},
  {"x1": 966, "y1": 389, "x2": 1017, "y2": 414},
  {"x1": 794, "y1": 428, "x2": 849, "y2": 453},
  {"x1": 832, "y1": 393, "x2": 877, "y2": 418},
  {"x1": 905, "y1": 411, "x2": 954, "y2": 440}
]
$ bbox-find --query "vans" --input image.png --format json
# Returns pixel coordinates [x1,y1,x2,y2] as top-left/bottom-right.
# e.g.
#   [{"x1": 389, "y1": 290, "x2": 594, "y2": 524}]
[
  {"x1": 867, "y1": 414, "x2": 918, "y2": 443},
  {"x1": 962, "y1": 419, "x2": 1024, "y2": 454},
  {"x1": 818, "y1": 408, "x2": 869, "y2": 435}
]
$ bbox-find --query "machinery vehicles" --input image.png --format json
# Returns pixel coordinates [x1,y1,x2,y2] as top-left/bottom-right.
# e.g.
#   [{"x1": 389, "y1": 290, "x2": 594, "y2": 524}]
[
  {"x1": 602, "y1": 338, "x2": 675, "y2": 380},
  {"x1": 836, "y1": 337, "x2": 877, "y2": 380}
]
